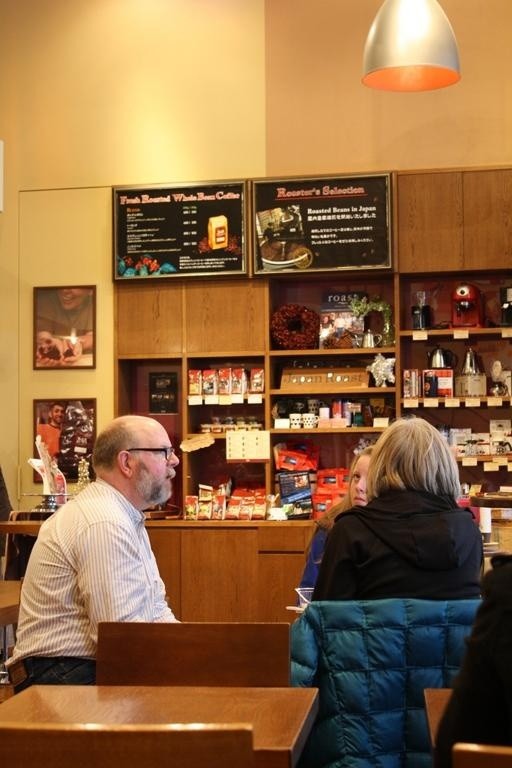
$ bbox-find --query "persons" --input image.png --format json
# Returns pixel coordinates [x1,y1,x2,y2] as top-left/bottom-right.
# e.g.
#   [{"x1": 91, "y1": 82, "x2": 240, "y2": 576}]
[
  {"x1": 430, "y1": 548, "x2": 511, "y2": 768},
  {"x1": 35, "y1": 402, "x2": 64, "y2": 458},
  {"x1": 295, "y1": 442, "x2": 379, "y2": 617},
  {"x1": 35, "y1": 288, "x2": 93, "y2": 366},
  {"x1": 5, "y1": 414, "x2": 179, "y2": 698},
  {"x1": 312, "y1": 415, "x2": 483, "y2": 599}
]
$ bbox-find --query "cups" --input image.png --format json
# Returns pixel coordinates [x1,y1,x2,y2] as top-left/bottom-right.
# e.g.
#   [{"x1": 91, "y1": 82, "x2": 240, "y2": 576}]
[
  {"x1": 289, "y1": 412, "x2": 303, "y2": 429},
  {"x1": 301, "y1": 413, "x2": 320, "y2": 430},
  {"x1": 307, "y1": 398, "x2": 325, "y2": 414}
]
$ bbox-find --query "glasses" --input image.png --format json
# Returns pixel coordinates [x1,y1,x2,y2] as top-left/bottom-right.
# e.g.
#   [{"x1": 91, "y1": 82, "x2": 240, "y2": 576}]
[{"x1": 126, "y1": 447, "x2": 175, "y2": 461}]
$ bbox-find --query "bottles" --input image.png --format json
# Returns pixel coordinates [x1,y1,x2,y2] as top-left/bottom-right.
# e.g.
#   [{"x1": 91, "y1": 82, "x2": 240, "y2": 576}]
[
  {"x1": 364, "y1": 405, "x2": 373, "y2": 426},
  {"x1": 424, "y1": 371, "x2": 437, "y2": 396},
  {"x1": 332, "y1": 397, "x2": 353, "y2": 426},
  {"x1": 410, "y1": 291, "x2": 432, "y2": 330}
]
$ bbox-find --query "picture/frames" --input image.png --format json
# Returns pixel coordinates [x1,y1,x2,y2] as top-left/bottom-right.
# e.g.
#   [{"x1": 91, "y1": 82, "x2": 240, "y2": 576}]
[
  {"x1": 32, "y1": 396, "x2": 98, "y2": 485},
  {"x1": 33, "y1": 285, "x2": 97, "y2": 371}
]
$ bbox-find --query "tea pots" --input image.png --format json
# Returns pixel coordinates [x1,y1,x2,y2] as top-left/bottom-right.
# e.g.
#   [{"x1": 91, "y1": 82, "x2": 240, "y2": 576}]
[
  {"x1": 460, "y1": 346, "x2": 481, "y2": 375},
  {"x1": 354, "y1": 328, "x2": 383, "y2": 348},
  {"x1": 427, "y1": 344, "x2": 457, "y2": 369}
]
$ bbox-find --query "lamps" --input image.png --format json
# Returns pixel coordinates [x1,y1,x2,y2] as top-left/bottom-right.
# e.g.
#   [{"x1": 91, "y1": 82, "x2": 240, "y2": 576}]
[{"x1": 361, "y1": 0, "x2": 462, "y2": 92}]
